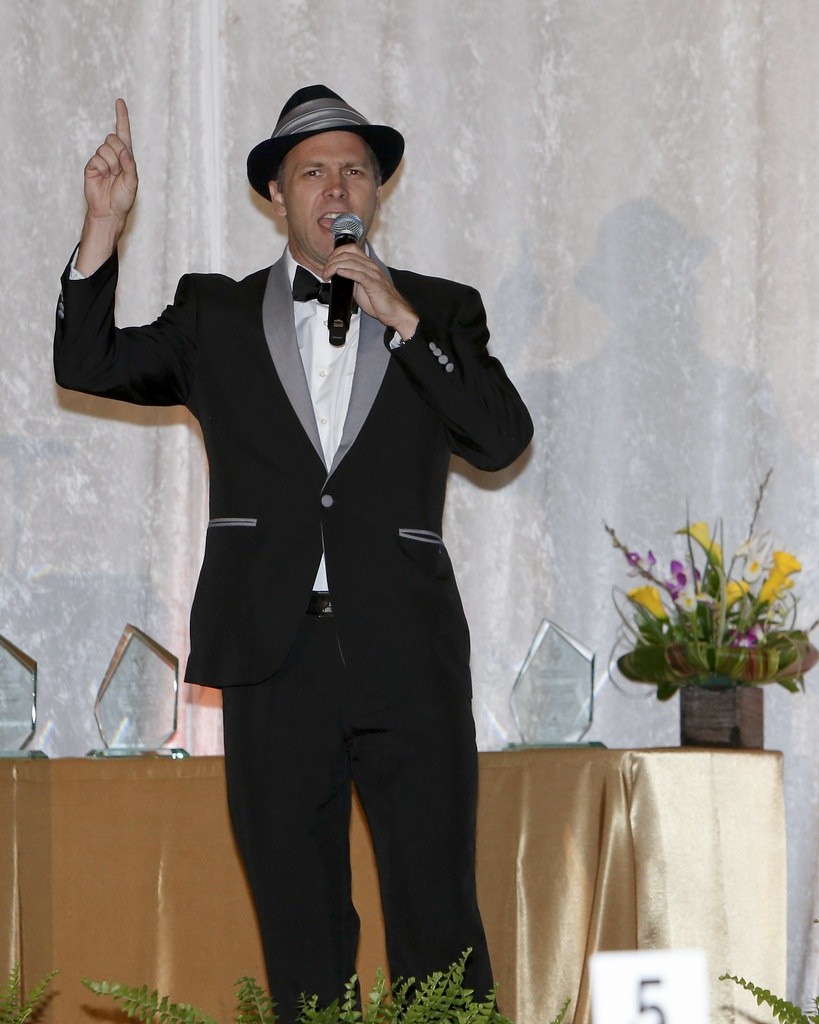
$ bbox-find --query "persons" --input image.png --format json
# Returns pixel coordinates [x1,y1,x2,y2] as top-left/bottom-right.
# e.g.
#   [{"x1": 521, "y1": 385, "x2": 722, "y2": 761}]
[{"x1": 53, "y1": 84, "x2": 535, "y2": 1024}]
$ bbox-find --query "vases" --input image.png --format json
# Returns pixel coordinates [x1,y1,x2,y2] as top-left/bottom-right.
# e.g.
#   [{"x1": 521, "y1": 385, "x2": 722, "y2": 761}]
[{"x1": 680, "y1": 686, "x2": 762, "y2": 749}]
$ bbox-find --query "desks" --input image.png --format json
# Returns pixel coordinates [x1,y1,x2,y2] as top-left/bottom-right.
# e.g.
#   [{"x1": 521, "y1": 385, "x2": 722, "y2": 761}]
[{"x1": 1, "y1": 746, "x2": 785, "y2": 1024}]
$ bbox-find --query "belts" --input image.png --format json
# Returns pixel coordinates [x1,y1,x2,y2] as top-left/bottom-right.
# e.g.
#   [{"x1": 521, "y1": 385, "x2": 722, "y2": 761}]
[{"x1": 306, "y1": 591, "x2": 334, "y2": 617}]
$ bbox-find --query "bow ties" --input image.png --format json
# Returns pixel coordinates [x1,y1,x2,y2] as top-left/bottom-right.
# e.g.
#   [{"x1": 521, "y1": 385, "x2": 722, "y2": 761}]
[{"x1": 292, "y1": 265, "x2": 359, "y2": 314}]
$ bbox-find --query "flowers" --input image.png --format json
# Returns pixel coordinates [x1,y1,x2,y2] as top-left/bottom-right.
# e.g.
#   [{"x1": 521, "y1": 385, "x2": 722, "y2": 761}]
[{"x1": 602, "y1": 469, "x2": 819, "y2": 702}]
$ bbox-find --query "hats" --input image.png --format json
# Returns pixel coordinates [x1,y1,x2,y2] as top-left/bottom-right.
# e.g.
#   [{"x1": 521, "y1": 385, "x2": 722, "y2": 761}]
[{"x1": 247, "y1": 83, "x2": 406, "y2": 203}]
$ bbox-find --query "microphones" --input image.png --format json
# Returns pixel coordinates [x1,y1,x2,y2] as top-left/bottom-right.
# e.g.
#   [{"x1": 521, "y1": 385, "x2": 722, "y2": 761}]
[{"x1": 328, "y1": 213, "x2": 364, "y2": 347}]
